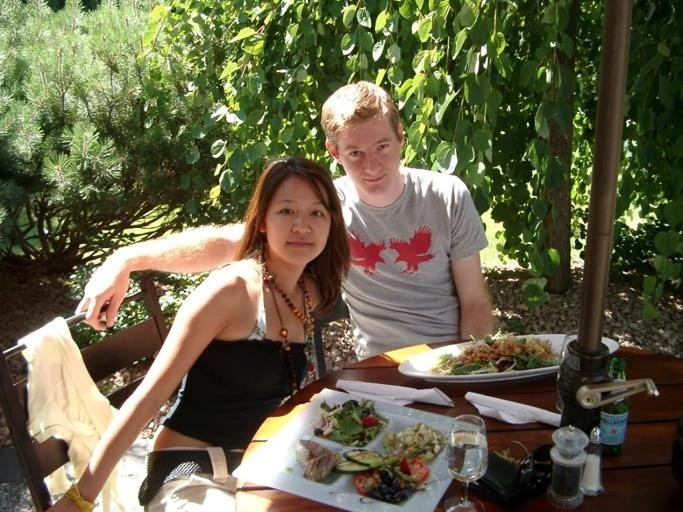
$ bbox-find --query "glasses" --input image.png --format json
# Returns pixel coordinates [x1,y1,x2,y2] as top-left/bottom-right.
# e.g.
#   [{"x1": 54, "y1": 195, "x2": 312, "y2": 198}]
[{"x1": 514, "y1": 444, "x2": 554, "y2": 496}]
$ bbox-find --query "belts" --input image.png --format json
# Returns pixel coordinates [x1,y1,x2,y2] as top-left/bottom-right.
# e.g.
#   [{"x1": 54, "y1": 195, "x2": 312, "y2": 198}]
[{"x1": 138, "y1": 449, "x2": 242, "y2": 505}]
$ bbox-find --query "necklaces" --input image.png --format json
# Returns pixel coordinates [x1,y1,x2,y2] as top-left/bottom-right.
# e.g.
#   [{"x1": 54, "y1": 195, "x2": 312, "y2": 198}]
[{"x1": 259, "y1": 241, "x2": 316, "y2": 396}]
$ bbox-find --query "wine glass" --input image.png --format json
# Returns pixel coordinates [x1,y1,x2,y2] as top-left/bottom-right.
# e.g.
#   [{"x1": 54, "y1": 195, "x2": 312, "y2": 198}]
[{"x1": 440, "y1": 413, "x2": 490, "y2": 512}]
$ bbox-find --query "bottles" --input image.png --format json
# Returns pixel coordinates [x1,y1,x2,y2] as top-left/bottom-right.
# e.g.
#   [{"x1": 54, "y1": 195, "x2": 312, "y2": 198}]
[
  {"x1": 544, "y1": 422, "x2": 590, "y2": 511},
  {"x1": 600, "y1": 355, "x2": 630, "y2": 457}
]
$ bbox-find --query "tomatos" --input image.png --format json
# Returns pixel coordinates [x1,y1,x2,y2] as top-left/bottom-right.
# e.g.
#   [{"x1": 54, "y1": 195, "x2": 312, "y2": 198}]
[{"x1": 351, "y1": 456, "x2": 429, "y2": 495}]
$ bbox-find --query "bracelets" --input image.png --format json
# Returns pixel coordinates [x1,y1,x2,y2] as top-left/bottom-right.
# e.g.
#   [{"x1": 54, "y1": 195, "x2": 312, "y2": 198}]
[{"x1": 64, "y1": 484, "x2": 94, "y2": 512}]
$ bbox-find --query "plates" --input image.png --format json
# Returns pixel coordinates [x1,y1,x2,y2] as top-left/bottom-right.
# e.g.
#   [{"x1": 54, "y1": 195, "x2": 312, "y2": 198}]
[
  {"x1": 396, "y1": 332, "x2": 621, "y2": 384},
  {"x1": 231, "y1": 386, "x2": 480, "y2": 512}
]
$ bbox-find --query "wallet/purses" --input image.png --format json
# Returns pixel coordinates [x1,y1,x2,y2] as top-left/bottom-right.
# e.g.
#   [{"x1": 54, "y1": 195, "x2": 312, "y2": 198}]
[{"x1": 463, "y1": 452, "x2": 519, "y2": 499}]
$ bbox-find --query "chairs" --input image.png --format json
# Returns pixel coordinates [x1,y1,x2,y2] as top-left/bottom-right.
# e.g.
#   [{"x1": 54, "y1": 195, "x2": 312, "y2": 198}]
[
  {"x1": 312, "y1": 296, "x2": 354, "y2": 378},
  {"x1": 2, "y1": 274, "x2": 182, "y2": 512}
]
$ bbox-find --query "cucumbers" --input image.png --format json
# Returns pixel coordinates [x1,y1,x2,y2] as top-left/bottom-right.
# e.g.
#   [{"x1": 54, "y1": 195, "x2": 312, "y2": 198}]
[{"x1": 334, "y1": 448, "x2": 385, "y2": 475}]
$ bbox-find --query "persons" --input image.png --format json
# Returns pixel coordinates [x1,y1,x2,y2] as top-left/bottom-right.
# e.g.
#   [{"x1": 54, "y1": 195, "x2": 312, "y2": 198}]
[
  {"x1": 46, "y1": 159, "x2": 350, "y2": 512},
  {"x1": 74, "y1": 81, "x2": 495, "y2": 364}
]
